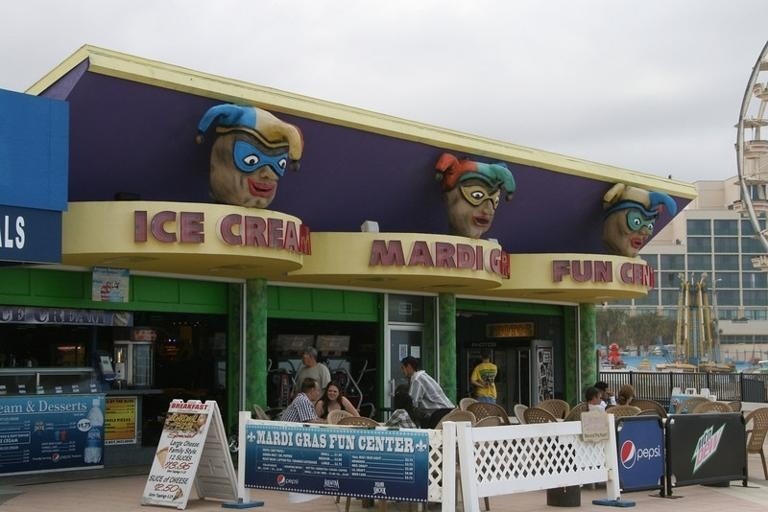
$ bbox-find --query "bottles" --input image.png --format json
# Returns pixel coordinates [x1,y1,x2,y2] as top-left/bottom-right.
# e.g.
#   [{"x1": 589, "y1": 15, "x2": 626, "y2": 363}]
[{"x1": 84, "y1": 399, "x2": 104, "y2": 464}]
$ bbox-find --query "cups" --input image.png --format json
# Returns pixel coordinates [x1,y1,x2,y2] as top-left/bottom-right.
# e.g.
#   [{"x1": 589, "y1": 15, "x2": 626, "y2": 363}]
[
  {"x1": 700, "y1": 388, "x2": 711, "y2": 396},
  {"x1": 671, "y1": 387, "x2": 682, "y2": 396}
]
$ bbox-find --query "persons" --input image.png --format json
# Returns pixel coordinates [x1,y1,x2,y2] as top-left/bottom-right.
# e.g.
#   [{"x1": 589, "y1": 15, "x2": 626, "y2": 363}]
[
  {"x1": 585, "y1": 388, "x2": 605, "y2": 411},
  {"x1": 592, "y1": 378, "x2": 618, "y2": 407},
  {"x1": 617, "y1": 382, "x2": 646, "y2": 416},
  {"x1": 433, "y1": 153, "x2": 517, "y2": 240},
  {"x1": 194, "y1": 103, "x2": 304, "y2": 208},
  {"x1": 469, "y1": 345, "x2": 500, "y2": 406},
  {"x1": 289, "y1": 348, "x2": 331, "y2": 406},
  {"x1": 281, "y1": 379, "x2": 326, "y2": 425},
  {"x1": 386, "y1": 394, "x2": 421, "y2": 430},
  {"x1": 401, "y1": 357, "x2": 456, "y2": 430},
  {"x1": 394, "y1": 384, "x2": 411, "y2": 395},
  {"x1": 600, "y1": 183, "x2": 678, "y2": 257},
  {"x1": 314, "y1": 382, "x2": 362, "y2": 419}
]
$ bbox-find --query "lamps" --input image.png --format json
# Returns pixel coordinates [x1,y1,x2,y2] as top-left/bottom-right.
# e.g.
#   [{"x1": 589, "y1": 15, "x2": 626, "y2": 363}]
[
  {"x1": 360, "y1": 220, "x2": 379, "y2": 233},
  {"x1": 486, "y1": 238, "x2": 499, "y2": 244}
]
